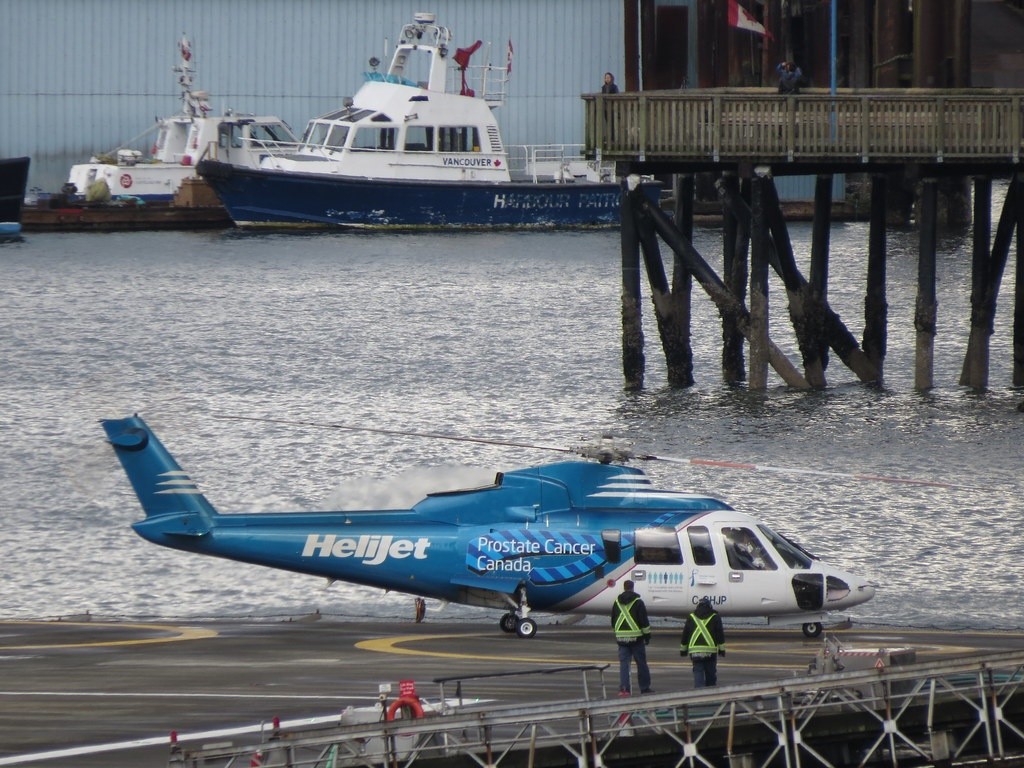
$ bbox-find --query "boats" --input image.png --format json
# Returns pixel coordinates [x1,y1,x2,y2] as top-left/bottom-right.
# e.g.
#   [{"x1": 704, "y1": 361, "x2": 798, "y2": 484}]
[
  {"x1": 18, "y1": 27, "x2": 301, "y2": 226},
  {"x1": 192, "y1": 11, "x2": 668, "y2": 234},
  {"x1": 97, "y1": 410, "x2": 1015, "y2": 637}
]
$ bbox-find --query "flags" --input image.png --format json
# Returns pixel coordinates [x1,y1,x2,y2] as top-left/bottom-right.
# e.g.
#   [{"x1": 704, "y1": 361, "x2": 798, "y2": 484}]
[
  {"x1": 508, "y1": 37, "x2": 514, "y2": 74},
  {"x1": 729, "y1": 0, "x2": 775, "y2": 41}
]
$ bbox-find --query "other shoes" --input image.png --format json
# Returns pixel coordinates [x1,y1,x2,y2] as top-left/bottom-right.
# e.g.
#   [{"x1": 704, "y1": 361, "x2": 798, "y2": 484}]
[{"x1": 640, "y1": 689, "x2": 654, "y2": 693}]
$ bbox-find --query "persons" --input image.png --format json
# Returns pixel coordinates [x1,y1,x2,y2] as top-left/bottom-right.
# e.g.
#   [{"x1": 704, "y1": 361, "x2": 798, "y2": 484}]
[
  {"x1": 732, "y1": 526, "x2": 766, "y2": 570},
  {"x1": 777, "y1": 60, "x2": 804, "y2": 138},
  {"x1": 611, "y1": 580, "x2": 657, "y2": 696},
  {"x1": 680, "y1": 598, "x2": 728, "y2": 689},
  {"x1": 601, "y1": 70, "x2": 622, "y2": 142}
]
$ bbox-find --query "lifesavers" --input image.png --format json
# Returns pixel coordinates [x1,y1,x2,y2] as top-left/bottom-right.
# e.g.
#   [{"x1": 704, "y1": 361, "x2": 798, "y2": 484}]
[{"x1": 388, "y1": 697, "x2": 424, "y2": 737}]
